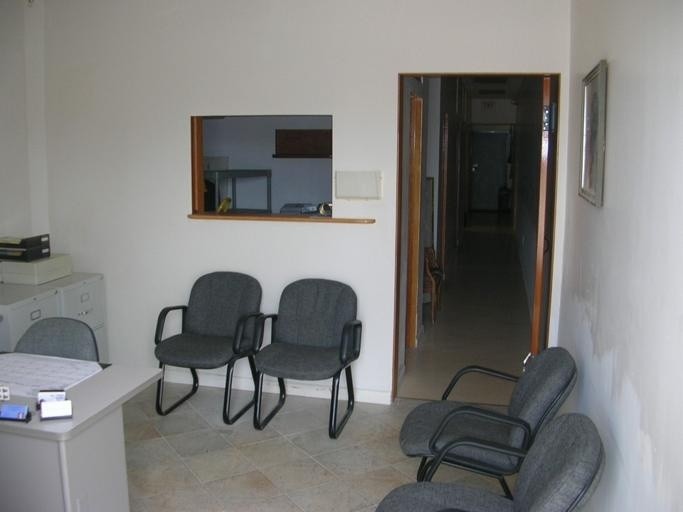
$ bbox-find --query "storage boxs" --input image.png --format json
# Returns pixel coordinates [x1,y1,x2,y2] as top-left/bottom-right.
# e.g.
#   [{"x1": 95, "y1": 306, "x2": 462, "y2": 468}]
[
  {"x1": 0, "y1": 282, "x2": 60, "y2": 354},
  {"x1": 37, "y1": 272, "x2": 109, "y2": 331},
  {"x1": 89, "y1": 325, "x2": 109, "y2": 364}
]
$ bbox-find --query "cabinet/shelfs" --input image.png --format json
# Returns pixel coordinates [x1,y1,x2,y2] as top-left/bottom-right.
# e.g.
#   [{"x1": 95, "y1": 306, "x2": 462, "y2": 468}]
[{"x1": 204, "y1": 168, "x2": 273, "y2": 215}]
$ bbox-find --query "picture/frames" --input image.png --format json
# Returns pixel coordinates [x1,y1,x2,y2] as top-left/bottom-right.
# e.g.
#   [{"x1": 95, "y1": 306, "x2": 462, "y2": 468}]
[{"x1": 577, "y1": 58, "x2": 609, "y2": 209}]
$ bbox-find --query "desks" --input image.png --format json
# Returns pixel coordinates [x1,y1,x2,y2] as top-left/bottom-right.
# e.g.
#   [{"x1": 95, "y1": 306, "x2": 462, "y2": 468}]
[{"x1": 0, "y1": 349, "x2": 163, "y2": 512}]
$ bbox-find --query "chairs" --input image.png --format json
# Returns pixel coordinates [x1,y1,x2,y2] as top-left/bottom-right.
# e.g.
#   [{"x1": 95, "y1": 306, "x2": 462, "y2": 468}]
[
  {"x1": 154, "y1": 272, "x2": 265, "y2": 424},
  {"x1": 423, "y1": 247, "x2": 443, "y2": 325},
  {"x1": 17, "y1": 316, "x2": 100, "y2": 361},
  {"x1": 374, "y1": 413, "x2": 607, "y2": 512},
  {"x1": 400, "y1": 346, "x2": 578, "y2": 501},
  {"x1": 250, "y1": 278, "x2": 362, "y2": 440}
]
